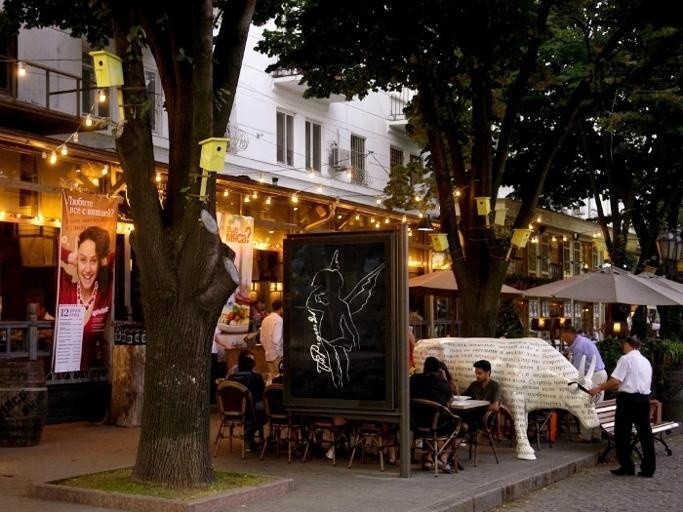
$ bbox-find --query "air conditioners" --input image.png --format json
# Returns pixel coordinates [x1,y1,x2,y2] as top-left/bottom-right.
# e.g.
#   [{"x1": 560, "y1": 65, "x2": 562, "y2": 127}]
[{"x1": 333, "y1": 147, "x2": 350, "y2": 168}]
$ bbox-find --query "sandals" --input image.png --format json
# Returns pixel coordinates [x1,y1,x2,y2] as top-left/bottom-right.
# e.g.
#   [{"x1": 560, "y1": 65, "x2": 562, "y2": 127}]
[{"x1": 424, "y1": 460, "x2": 453, "y2": 474}]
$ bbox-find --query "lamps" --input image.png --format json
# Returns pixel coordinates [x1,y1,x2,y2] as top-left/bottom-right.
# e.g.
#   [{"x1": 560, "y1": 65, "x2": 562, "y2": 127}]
[{"x1": 418, "y1": 214, "x2": 433, "y2": 230}]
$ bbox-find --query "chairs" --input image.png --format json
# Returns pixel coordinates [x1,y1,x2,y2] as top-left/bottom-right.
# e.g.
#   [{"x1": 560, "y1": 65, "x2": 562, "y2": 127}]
[{"x1": 595, "y1": 399, "x2": 680, "y2": 464}]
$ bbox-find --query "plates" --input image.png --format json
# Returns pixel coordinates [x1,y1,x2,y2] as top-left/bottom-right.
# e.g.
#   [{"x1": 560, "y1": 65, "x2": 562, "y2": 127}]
[
  {"x1": 453, "y1": 395, "x2": 472, "y2": 401},
  {"x1": 217, "y1": 323, "x2": 249, "y2": 333}
]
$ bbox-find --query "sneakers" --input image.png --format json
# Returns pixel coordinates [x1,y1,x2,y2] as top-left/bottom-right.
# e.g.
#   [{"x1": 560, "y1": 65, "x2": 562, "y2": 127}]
[{"x1": 324, "y1": 448, "x2": 334, "y2": 459}]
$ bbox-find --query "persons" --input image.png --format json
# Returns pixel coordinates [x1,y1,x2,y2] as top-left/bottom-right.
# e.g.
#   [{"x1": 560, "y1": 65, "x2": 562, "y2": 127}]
[
  {"x1": 589, "y1": 336, "x2": 656, "y2": 478},
  {"x1": 410, "y1": 357, "x2": 469, "y2": 473},
  {"x1": 460, "y1": 360, "x2": 502, "y2": 432},
  {"x1": 58, "y1": 226, "x2": 117, "y2": 367},
  {"x1": 225, "y1": 349, "x2": 291, "y2": 453},
  {"x1": 258, "y1": 299, "x2": 284, "y2": 376},
  {"x1": 211, "y1": 326, "x2": 236, "y2": 404},
  {"x1": 559, "y1": 325, "x2": 608, "y2": 442}
]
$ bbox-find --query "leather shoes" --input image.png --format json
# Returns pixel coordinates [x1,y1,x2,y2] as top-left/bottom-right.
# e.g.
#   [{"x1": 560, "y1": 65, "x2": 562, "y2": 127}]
[
  {"x1": 571, "y1": 435, "x2": 601, "y2": 443},
  {"x1": 609, "y1": 466, "x2": 654, "y2": 477}
]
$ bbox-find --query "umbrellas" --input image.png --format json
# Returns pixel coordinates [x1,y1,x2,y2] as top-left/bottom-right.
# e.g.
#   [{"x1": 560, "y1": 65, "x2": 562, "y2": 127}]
[
  {"x1": 635, "y1": 271, "x2": 683, "y2": 322},
  {"x1": 523, "y1": 267, "x2": 683, "y2": 335},
  {"x1": 409, "y1": 270, "x2": 524, "y2": 294}
]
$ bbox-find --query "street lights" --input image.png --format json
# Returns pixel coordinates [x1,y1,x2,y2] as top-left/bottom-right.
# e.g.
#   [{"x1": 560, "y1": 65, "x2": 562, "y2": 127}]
[{"x1": 656, "y1": 231, "x2": 683, "y2": 283}]
